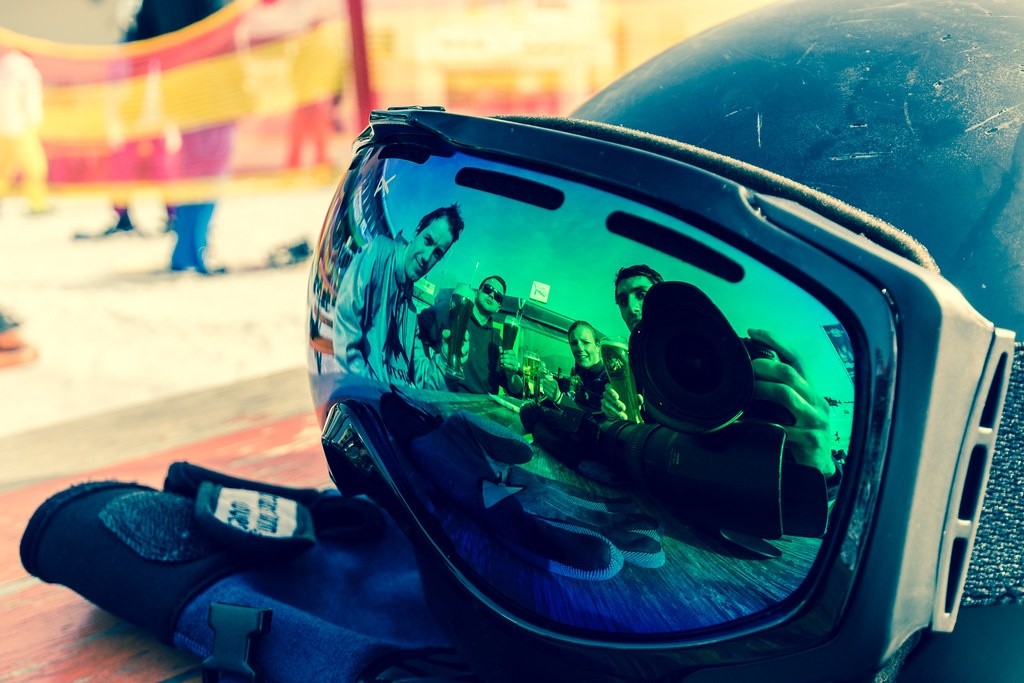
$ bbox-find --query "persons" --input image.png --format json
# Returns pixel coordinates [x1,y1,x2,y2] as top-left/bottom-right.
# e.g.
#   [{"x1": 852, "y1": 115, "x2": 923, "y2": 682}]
[
  {"x1": 121, "y1": 1, "x2": 252, "y2": 275},
  {"x1": 331, "y1": 201, "x2": 850, "y2": 541}
]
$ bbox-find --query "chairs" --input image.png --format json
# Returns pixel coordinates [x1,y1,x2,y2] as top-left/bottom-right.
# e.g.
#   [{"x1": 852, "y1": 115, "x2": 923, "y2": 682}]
[{"x1": 309, "y1": 259, "x2": 338, "y2": 376}]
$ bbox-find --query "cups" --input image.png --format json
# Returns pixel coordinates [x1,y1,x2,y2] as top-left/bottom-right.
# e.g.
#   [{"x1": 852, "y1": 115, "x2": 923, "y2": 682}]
[
  {"x1": 600, "y1": 336, "x2": 644, "y2": 425},
  {"x1": 523, "y1": 350, "x2": 554, "y2": 403},
  {"x1": 503, "y1": 315, "x2": 520, "y2": 350},
  {"x1": 443, "y1": 283, "x2": 476, "y2": 380}
]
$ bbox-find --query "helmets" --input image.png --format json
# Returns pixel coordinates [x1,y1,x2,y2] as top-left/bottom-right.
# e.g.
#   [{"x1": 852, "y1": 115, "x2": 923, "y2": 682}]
[{"x1": 576, "y1": 5, "x2": 1020, "y2": 683}]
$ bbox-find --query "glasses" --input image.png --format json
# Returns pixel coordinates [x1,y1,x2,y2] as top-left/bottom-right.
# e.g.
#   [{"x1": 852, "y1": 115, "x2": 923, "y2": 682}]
[
  {"x1": 482, "y1": 284, "x2": 504, "y2": 305},
  {"x1": 312, "y1": 103, "x2": 1017, "y2": 666}
]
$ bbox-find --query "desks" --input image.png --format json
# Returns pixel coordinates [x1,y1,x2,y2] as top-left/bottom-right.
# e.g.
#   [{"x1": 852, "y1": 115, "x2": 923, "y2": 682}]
[{"x1": 407, "y1": 387, "x2": 824, "y2": 636}]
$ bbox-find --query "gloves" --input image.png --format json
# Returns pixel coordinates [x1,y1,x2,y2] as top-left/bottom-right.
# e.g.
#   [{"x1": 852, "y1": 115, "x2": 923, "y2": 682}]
[
  {"x1": 19, "y1": 462, "x2": 490, "y2": 683},
  {"x1": 382, "y1": 387, "x2": 669, "y2": 580}
]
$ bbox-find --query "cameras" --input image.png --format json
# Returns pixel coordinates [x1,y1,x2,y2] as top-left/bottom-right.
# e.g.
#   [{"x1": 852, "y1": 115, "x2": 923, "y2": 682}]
[{"x1": 629, "y1": 280, "x2": 797, "y2": 435}]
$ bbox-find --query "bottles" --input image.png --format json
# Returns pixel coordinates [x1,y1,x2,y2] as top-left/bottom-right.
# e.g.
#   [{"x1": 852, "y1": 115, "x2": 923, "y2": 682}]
[{"x1": 1, "y1": 50, "x2": 49, "y2": 215}]
